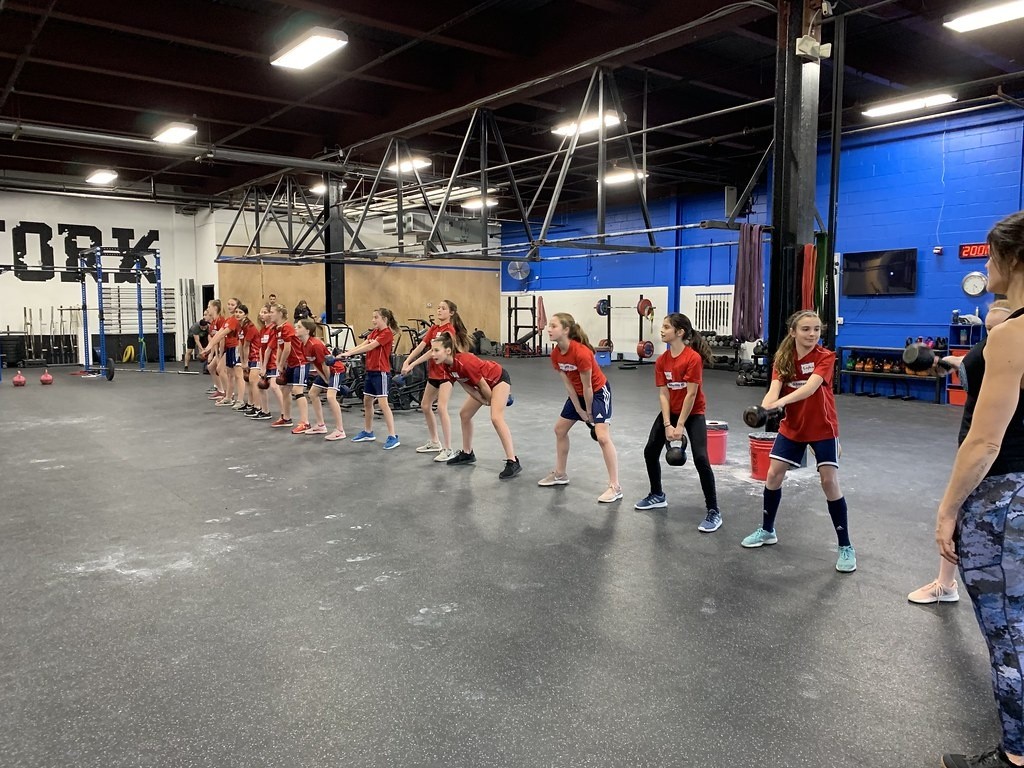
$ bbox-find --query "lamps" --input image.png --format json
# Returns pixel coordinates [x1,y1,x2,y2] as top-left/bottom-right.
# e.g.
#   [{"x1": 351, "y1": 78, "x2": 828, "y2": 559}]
[{"x1": 86, "y1": 2, "x2": 1024, "y2": 209}]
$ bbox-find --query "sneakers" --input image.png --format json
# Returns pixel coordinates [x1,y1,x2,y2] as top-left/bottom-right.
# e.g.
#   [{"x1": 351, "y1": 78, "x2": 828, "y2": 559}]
[
  {"x1": 697, "y1": 507, "x2": 723, "y2": 532},
  {"x1": 270, "y1": 414, "x2": 293, "y2": 427},
  {"x1": 434, "y1": 448, "x2": 454, "y2": 462},
  {"x1": 238, "y1": 403, "x2": 255, "y2": 411},
  {"x1": 215, "y1": 395, "x2": 235, "y2": 406},
  {"x1": 325, "y1": 430, "x2": 346, "y2": 440},
  {"x1": 447, "y1": 449, "x2": 476, "y2": 465},
  {"x1": 940, "y1": 743, "x2": 1014, "y2": 768},
  {"x1": 598, "y1": 484, "x2": 623, "y2": 502},
  {"x1": 835, "y1": 545, "x2": 856, "y2": 572},
  {"x1": 250, "y1": 410, "x2": 272, "y2": 420},
  {"x1": 305, "y1": 425, "x2": 328, "y2": 435},
  {"x1": 634, "y1": 492, "x2": 668, "y2": 510},
  {"x1": 231, "y1": 400, "x2": 245, "y2": 410},
  {"x1": 538, "y1": 470, "x2": 570, "y2": 486},
  {"x1": 206, "y1": 387, "x2": 218, "y2": 393},
  {"x1": 741, "y1": 528, "x2": 778, "y2": 547},
  {"x1": 383, "y1": 435, "x2": 401, "y2": 449},
  {"x1": 208, "y1": 391, "x2": 226, "y2": 399},
  {"x1": 499, "y1": 456, "x2": 522, "y2": 479},
  {"x1": 416, "y1": 440, "x2": 442, "y2": 452},
  {"x1": 351, "y1": 430, "x2": 375, "y2": 442},
  {"x1": 244, "y1": 407, "x2": 262, "y2": 416},
  {"x1": 292, "y1": 422, "x2": 311, "y2": 434},
  {"x1": 908, "y1": 578, "x2": 960, "y2": 604}
]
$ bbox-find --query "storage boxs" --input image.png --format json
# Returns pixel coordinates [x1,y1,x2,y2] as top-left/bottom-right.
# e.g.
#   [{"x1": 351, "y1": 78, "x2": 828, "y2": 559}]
[
  {"x1": 950, "y1": 348, "x2": 970, "y2": 384},
  {"x1": 946, "y1": 388, "x2": 968, "y2": 405}
]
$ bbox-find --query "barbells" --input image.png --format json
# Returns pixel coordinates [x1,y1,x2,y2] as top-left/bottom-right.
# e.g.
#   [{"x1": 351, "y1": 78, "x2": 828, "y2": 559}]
[
  {"x1": 599, "y1": 339, "x2": 654, "y2": 358},
  {"x1": 89, "y1": 358, "x2": 201, "y2": 382},
  {"x1": 593, "y1": 299, "x2": 656, "y2": 316}
]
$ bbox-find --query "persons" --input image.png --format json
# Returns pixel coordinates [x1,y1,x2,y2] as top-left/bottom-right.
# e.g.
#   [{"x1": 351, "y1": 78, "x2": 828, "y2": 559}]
[
  {"x1": 741, "y1": 309, "x2": 857, "y2": 572},
  {"x1": 538, "y1": 312, "x2": 623, "y2": 502},
  {"x1": 182, "y1": 320, "x2": 210, "y2": 371},
  {"x1": 633, "y1": 312, "x2": 723, "y2": 532},
  {"x1": 317, "y1": 311, "x2": 326, "y2": 324},
  {"x1": 908, "y1": 299, "x2": 1012, "y2": 603},
  {"x1": 251, "y1": 304, "x2": 283, "y2": 422},
  {"x1": 200, "y1": 298, "x2": 245, "y2": 409},
  {"x1": 294, "y1": 300, "x2": 317, "y2": 324},
  {"x1": 295, "y1": 319, "x2": 346, "y2": 441},
  {"x1": 269, "y1": 294, "x2": 276, "y2": 303},
  {"x1": 921, "y1": 212, "x2": 1024, "y2": 768},
  {"x1": 430, "y1": 331, "x2": 522, "y2": 478},
  {"x1": 270, "y1": 303, "x2": 311, "y2": 433},
  {"x1": 234, "y1": 304, "x2": 262, "y2": 416},
  {"x1": 401, "y1": 299, "x2": 467, "y2": 462},
  {"x1": 338, "y1": 308, "x2": 402, "y2": 450}
]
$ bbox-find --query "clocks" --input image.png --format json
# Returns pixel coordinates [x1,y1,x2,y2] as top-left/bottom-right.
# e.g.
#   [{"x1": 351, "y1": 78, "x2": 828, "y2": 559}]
[{"x1": 963, "y1": 272, "x2": 988, "y2": 296}]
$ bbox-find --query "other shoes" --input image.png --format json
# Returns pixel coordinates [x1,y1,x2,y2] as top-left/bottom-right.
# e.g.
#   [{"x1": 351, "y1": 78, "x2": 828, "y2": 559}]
[{"x1": 184, "y1": 368, "x2": 189, "y2": 372}]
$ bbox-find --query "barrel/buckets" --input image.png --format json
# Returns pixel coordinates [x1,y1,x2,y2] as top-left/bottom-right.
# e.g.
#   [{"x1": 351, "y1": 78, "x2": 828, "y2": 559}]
[
  {"x1": 747, "y1": 431, "x2": 778, "y2": 481},
  {"x1": 705, "y1": 420, "x2": 728, "y2": 466}
]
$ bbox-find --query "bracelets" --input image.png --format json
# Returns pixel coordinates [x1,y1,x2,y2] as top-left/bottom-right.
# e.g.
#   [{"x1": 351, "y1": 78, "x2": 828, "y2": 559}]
[{"x1": 665, "y1": 425, "x2": 670, "y2": 428}]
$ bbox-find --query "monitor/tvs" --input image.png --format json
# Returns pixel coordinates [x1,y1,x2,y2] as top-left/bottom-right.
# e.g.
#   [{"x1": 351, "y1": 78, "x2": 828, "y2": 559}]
[{"x1": 842, "y1": 248, "x2": 917, "y2": 295}]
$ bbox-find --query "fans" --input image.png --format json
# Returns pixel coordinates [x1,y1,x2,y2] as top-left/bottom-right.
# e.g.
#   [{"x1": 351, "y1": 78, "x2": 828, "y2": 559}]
[{"x1": 508, "y1": 260, "x2": 530, "y2": 292}]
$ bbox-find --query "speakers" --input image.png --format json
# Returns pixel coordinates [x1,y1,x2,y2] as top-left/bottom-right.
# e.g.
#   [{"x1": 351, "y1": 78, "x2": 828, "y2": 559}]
[{"x1": 724, "y1": 186, "x2": 737, "y2": 218}]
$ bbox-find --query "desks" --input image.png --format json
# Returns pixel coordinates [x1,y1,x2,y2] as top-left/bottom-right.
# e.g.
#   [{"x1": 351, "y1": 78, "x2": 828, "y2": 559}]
[{"x1": 838, "y1": 344, "x2": 949, "y2": 404}]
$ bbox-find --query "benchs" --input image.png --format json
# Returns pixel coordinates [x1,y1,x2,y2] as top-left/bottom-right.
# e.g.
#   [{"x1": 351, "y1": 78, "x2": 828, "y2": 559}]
[{"x1": 839, "y1": 369, "x2": 943, "y2": 404}]
